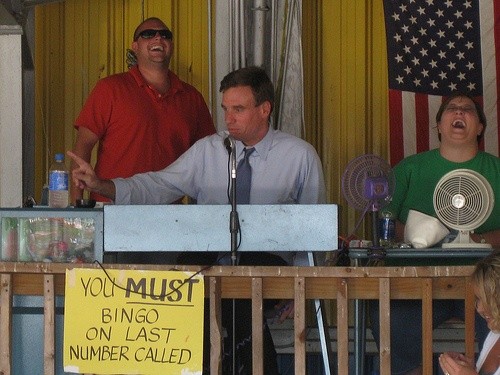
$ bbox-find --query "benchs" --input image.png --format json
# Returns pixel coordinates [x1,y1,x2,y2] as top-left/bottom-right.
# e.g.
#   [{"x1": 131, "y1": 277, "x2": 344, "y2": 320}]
[{"x1": 220, "y1": 317, "x2": 480, "y2": 375}]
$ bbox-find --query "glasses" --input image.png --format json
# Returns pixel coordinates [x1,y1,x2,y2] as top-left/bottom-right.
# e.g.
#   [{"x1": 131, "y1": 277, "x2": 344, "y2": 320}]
[
  {"x1": 134, "y1": 29, "x2": 173, "y2": 42},
  {"x1": 442, "y1": 104, "x2": 478, "y2": 117}
]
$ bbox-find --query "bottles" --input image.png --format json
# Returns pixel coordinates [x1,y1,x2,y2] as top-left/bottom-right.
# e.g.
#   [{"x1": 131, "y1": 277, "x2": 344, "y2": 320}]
[
  {"x1": 378, "y1": 196, "x2": 397, "y2": 250},
  {"x1": 348, "y1": 247, "x2": 387, "y2": 267},
  {"x1": 48, "y1": 153, "x2": 68, "y2": 209}
]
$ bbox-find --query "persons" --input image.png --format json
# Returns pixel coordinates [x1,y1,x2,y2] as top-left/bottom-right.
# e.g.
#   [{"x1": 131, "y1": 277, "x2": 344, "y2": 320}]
[
  {"x1": 69, "y1": 16, "x2": 217, "y2": 207},
  {"x1": 368, "y1": 90, "x2": 500, "y2": 375},
  {"x1": 68, "y1": 65, "x2": 326, "y2": 375},
  {"x1": 438, "y1": 245, "x2": 500, "y2": 375}
]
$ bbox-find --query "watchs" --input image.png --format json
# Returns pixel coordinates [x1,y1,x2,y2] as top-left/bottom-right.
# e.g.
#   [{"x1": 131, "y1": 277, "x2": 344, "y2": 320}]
[{"x1": 480, "y1": 234, "x2": 485, "y2": 244}]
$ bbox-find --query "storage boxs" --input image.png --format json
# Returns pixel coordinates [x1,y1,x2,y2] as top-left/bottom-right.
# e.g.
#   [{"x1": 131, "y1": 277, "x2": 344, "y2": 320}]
[{"x1": 1, "y1": 209, "x2": 104, "y2": 264}]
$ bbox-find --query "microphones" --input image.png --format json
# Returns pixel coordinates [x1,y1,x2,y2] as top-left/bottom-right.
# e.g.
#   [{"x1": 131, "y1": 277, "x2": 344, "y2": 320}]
[{"x1": 223, "y1": 135, "x2": 237, "y2": 174}]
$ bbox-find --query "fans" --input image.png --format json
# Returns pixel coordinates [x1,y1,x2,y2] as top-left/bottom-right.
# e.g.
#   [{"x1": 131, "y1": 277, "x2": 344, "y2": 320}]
[
  {"x1": 432, "y1": 169, "x2": 494, "y2": 249},
  {"x1": 341, "y1": 154, "x2": 396, "y2": 246}
]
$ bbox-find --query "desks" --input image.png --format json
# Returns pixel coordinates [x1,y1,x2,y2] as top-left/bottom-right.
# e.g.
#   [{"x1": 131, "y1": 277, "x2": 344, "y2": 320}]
[{"x1": 0, "y1": 260, "x2": 481, "y2": 375}]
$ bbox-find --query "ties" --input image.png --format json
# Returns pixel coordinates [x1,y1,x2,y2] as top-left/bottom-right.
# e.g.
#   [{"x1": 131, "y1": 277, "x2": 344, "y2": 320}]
[{"x1": 215, "y1": 147, "x2": 256, "y2": 266}]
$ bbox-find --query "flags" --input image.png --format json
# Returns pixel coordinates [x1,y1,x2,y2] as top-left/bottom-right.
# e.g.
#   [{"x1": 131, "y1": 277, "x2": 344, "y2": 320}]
[{"x1": 382, "y1": 0, "x2": 500, "y2": 168}]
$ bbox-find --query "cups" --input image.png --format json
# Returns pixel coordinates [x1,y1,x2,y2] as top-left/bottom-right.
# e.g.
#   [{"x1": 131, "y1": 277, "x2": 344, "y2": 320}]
[{"x1": 76, "y1": 199, "x2": 96, "y2": 209}]
